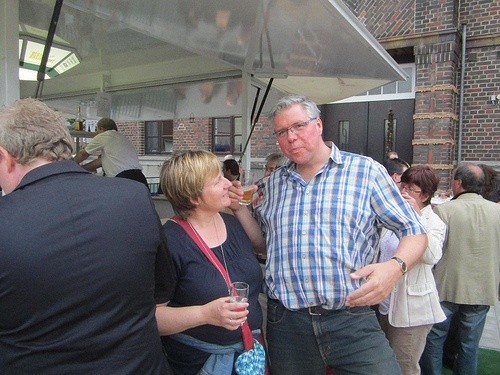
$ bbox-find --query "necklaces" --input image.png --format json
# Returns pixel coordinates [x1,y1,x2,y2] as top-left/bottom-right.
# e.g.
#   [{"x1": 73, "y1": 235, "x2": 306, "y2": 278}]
[{"x1": 186, "y1": 215, "x2": 231, "y2": 294}]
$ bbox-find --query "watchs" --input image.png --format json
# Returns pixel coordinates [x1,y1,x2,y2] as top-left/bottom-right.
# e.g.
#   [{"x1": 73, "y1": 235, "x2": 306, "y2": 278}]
[{"x1": 392, "y1": 257, "x2": 408, "y2": 276}]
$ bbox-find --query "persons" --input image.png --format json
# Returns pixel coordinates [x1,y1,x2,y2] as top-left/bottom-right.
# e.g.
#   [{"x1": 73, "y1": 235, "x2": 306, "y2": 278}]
[
  {"x1": 0, "y1": 98, "x2": 162, "y2": 375},
  {"x1": 154, "y1": 149, "x2": 271, "y2": 375},
  {"x1": 73, "y1": 118, "x2": 149, "y2": 191},
  {"x1": 221, "y1": 159, "x2": 240, "y2": 182},
  {"x1": 372, "y1": 157, "x2": 500, "y2": 375},
  {"x1": 263, "y1": 153, "x2": 289, "y2": 178},
  {"x1": 228, "y1": 94, "x2": 429, "y2": 375}
]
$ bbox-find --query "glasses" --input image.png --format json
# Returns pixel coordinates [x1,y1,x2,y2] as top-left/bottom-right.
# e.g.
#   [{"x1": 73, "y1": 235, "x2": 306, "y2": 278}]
[
  {"x1": 273, "y1": 117, "x2": 316, "y2": 140},
  {"x1": 398, "y1": 185, "x2": 423, "y2": 194}
]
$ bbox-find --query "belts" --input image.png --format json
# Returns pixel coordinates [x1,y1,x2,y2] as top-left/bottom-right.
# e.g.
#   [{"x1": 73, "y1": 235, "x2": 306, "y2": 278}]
[{"x1": 301, "y1": 306, "x2": 331, "y2": 317}]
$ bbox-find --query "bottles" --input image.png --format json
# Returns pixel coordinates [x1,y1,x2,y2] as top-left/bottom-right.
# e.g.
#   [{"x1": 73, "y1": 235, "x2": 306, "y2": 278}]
[{"x1": 74, "y1": 106, "x2": 82, "y2": 131}]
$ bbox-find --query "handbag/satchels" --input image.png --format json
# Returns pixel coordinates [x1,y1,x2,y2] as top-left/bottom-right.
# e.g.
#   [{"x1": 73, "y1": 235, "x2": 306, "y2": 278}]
[{"x1": 234, "y1": 329, "x2": 269, "y2": 375}]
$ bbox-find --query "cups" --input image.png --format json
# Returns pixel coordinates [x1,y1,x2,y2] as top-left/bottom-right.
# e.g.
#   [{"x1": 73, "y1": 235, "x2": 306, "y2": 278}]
[
  {"x1": 230, "y1": 282, "x2": 249, "y2": 313},
  {"x1": 238, "y1": 169, "x2": 257, "y2": 206}
]
